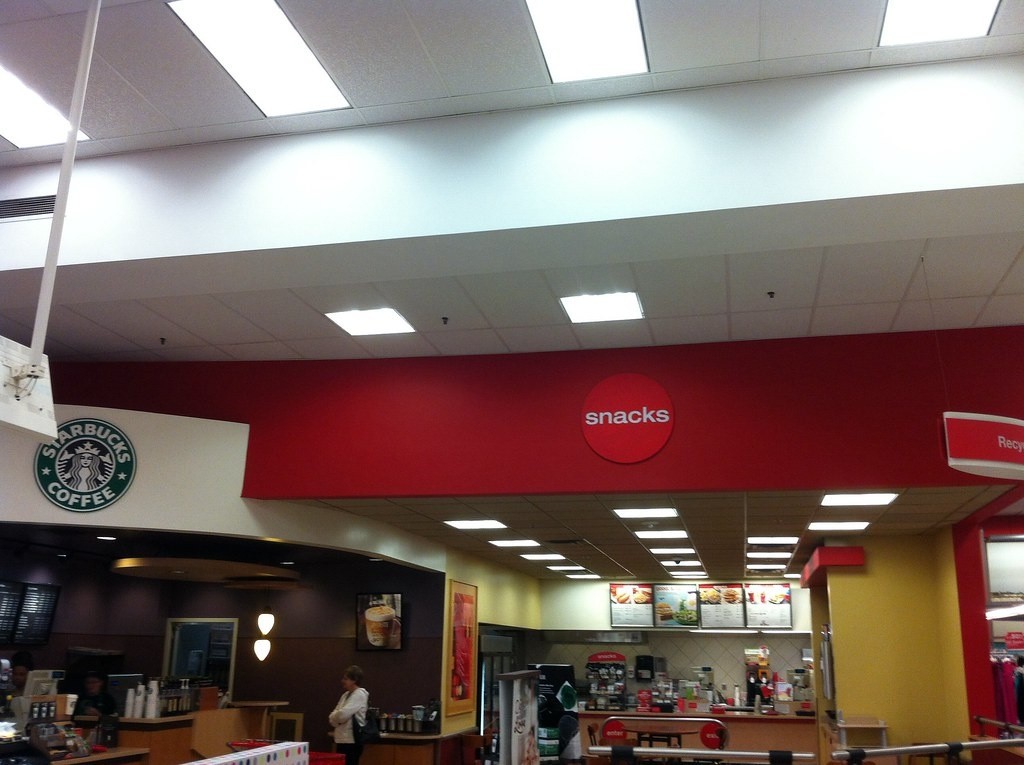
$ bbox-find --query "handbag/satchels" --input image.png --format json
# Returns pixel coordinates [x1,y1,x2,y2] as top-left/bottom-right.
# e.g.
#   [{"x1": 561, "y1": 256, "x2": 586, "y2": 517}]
[{"x1": 347, "y1": 688, "x2": 380, "y2": 744}]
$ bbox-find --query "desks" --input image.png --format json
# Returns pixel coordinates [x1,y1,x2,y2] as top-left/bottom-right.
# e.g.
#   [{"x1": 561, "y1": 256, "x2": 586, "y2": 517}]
[{"x1": 621, "y1": 725, "x2": 699, "y2": 762}]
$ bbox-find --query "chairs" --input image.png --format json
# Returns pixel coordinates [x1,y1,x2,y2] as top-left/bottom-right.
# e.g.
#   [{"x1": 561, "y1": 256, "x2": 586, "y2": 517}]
[
  {"x1": 693, "y1": 727, "x2": 728, "y2": 763},
  {"x1": 588, "y1": 723, "x2": 639, "y2": 765}
]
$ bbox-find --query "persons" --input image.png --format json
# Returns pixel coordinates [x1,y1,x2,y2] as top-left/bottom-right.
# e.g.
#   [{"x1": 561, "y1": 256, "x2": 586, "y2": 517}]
[
  {"x1": 47, "y1": 663, "x2": 87, "y2": 715},
  {"x1": 11, "y1": 651, "x2": 33, "y2": 686},
  {"x1": 329, "y1": 665, "x2": 370, "y2": 765},
  {"x1": 1014, "y1": 656, "x2": 1024, "y2": 725},
  {"x1": 78, "y1": 667, "x2": 118, "y2": 715}
]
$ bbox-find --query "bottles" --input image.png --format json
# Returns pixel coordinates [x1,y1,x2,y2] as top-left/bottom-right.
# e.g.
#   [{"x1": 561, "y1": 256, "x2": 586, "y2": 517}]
[
  {"x1": 754, "y1": 692, "x2": 761, "y2": 715},
  {"x1": 733, "y1": 684, "x2": 740, "y2": 706}
]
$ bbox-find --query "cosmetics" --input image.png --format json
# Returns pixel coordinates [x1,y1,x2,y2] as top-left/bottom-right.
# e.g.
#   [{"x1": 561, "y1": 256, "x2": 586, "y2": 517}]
[
  {"x1": 124, "y1": 678, "x2": 191, "y2": 719},
  {"x1": 32, "y1": 700, "x2": 56, "y2": 718}
]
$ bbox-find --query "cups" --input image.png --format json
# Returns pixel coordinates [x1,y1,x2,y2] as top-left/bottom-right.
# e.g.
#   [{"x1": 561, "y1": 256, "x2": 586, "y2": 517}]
[
  {"x1": 761, "y1": 594, "x2": 765, "y2": 603},
  {"x1": 379, "y1": 717, "x2": 424, "y2": 733},
  {"x1": 412, "y1": 707, "x2": 424, "y2": 720},
  {"x1": 748, "y1": 592, "x2": 754, "y2": 603},
  {"x1": 364, "y1": 607, "x2": 395, "y2": 646},
  {"x1": 124, "y1": 680, "x2": 158, "y2": 718},
  {"x1": 65, "y1": 694, "x2": 78, "y2": 715}
]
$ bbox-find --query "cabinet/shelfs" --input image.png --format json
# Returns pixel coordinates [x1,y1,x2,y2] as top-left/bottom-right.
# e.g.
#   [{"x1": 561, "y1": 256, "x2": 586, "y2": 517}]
[{"x1": 586, "y1": 652, "x2": 628, "y2": 711}]
